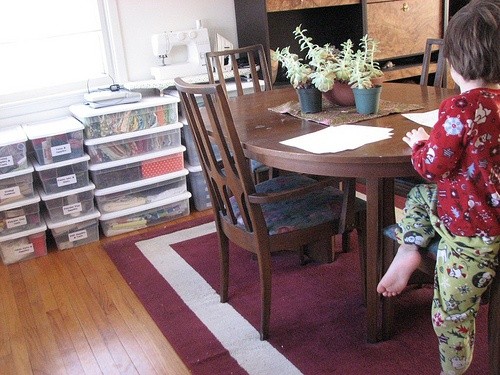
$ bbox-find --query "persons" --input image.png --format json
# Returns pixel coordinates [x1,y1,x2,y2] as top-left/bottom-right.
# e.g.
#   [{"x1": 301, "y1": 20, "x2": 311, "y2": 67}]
[{"x1": 374, "y1": 0, "x2": 500, "y2": 375}]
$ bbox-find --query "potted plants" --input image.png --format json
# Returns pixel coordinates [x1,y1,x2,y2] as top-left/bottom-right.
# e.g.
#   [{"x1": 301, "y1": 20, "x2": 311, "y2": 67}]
[{"x1": 272, "y1": 24, "x2": 385, "y2": 115}]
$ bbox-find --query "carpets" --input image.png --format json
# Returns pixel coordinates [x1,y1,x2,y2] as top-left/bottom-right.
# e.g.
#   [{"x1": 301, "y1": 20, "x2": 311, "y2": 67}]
[{"x1": 101, "y1": 185, "x2": 500, "y2": 375}]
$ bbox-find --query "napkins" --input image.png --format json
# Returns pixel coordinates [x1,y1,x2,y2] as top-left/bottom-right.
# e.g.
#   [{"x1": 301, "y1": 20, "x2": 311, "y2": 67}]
[
  {"x1": 402, "y1": 108, "x2": 441, "y2": 128},
  {"x1": 279, "y1": 123, "x2": 397, "y2": 153}
]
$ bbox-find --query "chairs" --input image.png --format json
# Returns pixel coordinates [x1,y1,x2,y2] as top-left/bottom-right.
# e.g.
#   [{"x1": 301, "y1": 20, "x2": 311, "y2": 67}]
[{"x1": 177, "y1": 44, "x2": 500, "y2": 375}]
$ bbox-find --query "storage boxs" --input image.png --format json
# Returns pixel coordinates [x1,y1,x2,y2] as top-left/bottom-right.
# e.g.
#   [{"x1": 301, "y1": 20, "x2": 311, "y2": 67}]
[{"x1": 0, "y1": 80, "x2": 267, "y2": 266}]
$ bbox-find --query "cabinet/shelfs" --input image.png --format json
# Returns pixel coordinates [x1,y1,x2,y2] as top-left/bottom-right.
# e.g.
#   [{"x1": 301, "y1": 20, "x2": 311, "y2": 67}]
[{"x1": 234, "y1": 0, "x2": 452, "y2": 87}]
